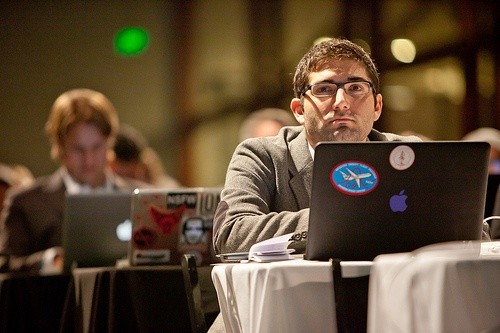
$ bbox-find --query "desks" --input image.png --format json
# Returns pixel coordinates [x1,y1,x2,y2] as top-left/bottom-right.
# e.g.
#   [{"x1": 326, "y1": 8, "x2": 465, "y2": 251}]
[{"x1": 0, "y1": 240, "x2": 500, "y2": 333}]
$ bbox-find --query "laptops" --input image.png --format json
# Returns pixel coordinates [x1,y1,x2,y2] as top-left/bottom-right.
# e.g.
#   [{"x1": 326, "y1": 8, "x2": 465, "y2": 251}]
[
  {"x1": 305, "y1": 141, "x2": 492, "y2": 260},
  {"x1": 59, "y1": 186, "x2": 134, "y2": 275},
  {"x1": 117, "y1": 187, "x2": 222, "y2": 266}
]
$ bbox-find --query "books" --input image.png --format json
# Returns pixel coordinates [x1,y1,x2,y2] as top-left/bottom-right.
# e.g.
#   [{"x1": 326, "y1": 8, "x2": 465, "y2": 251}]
[{"x1": 215, "y1": 232, "x2": 295, "y2": 263}]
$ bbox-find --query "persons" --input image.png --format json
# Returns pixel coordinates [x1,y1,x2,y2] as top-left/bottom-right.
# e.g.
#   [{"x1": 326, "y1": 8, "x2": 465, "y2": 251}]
[
  {"x1": 0, "y1": 87, "x2": 160, "y2": 279},
  {"x1": 461, "y1": 126, "x2": 500, "y2": 176},
  {"x1": 108, "y1": 123, "x2": 182, "y2": 188},
  {"x1": 236, "y1": 106, "x2": 299, "y2": 142},
  {"x1": 210, "y1": 35, "x2": 430, "y2": 256},
  {"x1": 0, "y1": 162, "x2": 37, "y2": 215}
]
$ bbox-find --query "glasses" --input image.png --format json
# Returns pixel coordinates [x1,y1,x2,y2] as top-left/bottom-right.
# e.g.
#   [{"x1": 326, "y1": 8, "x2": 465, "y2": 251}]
[{"x1": 302, "y1": 81, "x2": 375, "y2": 98}]
